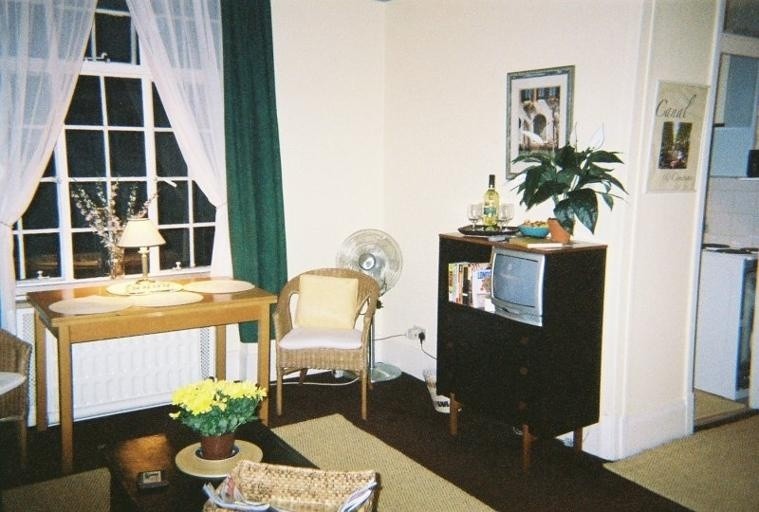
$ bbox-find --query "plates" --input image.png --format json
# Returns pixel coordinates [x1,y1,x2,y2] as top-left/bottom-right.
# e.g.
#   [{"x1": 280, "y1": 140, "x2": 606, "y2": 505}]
[{"x1": 457, "y1": 224, "x2": 521, "y2": 234}]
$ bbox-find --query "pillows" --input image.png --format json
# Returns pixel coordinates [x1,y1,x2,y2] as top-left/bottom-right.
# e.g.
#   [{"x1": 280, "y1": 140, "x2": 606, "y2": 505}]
[{"x1": 293, "y1": 271, "x2": 360, "y2": 332}]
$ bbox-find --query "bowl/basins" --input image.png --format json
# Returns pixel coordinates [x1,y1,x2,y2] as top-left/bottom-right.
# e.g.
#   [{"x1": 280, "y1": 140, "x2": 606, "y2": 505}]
[{"x1": 517, "y1": 224, "x2": 549, "y2": 238}]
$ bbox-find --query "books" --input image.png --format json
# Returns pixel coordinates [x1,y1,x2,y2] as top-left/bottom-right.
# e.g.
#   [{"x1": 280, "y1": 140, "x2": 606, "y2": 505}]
[
  {"x1": 202, "y1": 470, "x2": 289, "y2": 512},
  {"x1": 508, "y1": 235, "x2": 564, "y2": 249},
  {"x1": 447, "y1": 259, "x2": 495, "y2": 312},
  {"x1": 339, "y1": 480, "x2": 379, "y2": 512}
]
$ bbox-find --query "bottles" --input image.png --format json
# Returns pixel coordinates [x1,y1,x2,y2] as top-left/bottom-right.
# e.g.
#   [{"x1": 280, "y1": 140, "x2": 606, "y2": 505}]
[{"x1": 482, "y1": 174, "x2": 500, "y2": 227}]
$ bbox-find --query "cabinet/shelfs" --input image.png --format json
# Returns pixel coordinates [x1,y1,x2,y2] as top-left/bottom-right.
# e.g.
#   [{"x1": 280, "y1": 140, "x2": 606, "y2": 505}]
[{"x1": 433, "y1": 230, "x2": 608, "y2": 476}]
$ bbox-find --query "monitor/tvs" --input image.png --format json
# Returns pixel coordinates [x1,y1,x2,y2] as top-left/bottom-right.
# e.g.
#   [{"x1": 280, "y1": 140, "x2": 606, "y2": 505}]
[{"x1": 489, "y1": 249, "x2": 544, "y2": 327}]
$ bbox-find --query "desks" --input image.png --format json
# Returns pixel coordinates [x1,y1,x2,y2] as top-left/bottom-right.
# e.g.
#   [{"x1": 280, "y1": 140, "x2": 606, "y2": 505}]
[
  {"x1": 104, "y1": 419, "x2": 360, "y2": 511},
  {"x1": 23, "y1": 275, "x2": 279, "y2": 476}
]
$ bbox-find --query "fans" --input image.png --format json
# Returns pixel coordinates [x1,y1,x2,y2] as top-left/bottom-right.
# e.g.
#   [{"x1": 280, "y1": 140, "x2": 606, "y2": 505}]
[{"x1": 334, "y1": 227, "x2": 405, "y2": 383}]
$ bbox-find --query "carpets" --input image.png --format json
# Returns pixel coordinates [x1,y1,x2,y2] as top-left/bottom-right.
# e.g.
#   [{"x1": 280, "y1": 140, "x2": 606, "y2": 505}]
[
  {"x1": 695, "y1": 386, "x2": 748, "y2": 420},
  {"x1": 601, "y1": 413, "x2": 758, "y2": 511},
  {"x1": 0, "y1": 411, "x2": 500, "y2": 511}
]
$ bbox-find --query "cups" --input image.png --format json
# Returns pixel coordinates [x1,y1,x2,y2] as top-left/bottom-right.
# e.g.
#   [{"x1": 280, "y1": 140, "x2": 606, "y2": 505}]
[{"x1": 746, "y1": 149, "x2": 759, "y2": 176}]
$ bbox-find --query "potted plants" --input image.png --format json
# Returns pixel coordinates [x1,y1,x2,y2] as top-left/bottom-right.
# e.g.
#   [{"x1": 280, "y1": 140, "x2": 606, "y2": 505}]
[{"x1": 507, "y1": 135, "x2": 632, "y2": 245}]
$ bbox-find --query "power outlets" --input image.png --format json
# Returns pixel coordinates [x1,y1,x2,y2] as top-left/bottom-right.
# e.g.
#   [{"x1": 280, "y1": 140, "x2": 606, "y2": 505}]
[{"x1": 412, "y1": 325, "x2": 426, "y2": 340}]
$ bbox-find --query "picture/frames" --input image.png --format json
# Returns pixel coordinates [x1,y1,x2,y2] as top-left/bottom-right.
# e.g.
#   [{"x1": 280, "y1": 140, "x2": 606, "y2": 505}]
[{"x1": 505, "y1": 62, "x2": 575, "y2": 182}]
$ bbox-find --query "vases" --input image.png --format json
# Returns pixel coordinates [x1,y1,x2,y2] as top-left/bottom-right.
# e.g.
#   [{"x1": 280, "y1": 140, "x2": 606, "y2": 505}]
[
  {"x1": 197, "y1": 432, "x2": 238, "y2": 460},
  {"x1": 98, "y1": 231, "x2": 128, "y2": 280}
]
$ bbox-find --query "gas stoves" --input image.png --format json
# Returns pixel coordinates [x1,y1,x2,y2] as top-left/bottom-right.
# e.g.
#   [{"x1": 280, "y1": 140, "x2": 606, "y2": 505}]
[{"x1": 701, "y1": 243, "x2": 759, "y2": 255}]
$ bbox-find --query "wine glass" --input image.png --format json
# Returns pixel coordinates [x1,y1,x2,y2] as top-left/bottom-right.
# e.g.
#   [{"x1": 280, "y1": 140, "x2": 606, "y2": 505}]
[{"x1": 466, "y1": 201, "x2": 515, "y2": 232}]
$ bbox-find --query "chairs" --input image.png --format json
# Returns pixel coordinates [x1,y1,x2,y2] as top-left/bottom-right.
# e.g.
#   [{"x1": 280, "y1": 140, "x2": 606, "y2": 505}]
[
  {"x1": 0, "y1": 326, "x2": 34, "y2": 475},
  {"x1": 272, "y1": 267, "x2": 382, "y2": 421}
]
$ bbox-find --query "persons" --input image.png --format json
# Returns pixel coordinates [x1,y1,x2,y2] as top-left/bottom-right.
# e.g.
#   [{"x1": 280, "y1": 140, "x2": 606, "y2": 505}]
[
  {"x1": 522, "y1": 101, "x2": 538, "y2": 132},
  {"x1": 532, "y1": 113, "x2": 548, "y2": 143}
]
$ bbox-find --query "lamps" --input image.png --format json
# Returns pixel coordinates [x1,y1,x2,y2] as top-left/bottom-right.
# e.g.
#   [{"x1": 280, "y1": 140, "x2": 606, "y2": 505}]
[{"x1": 115, "y1": 216, "x2": 168, "y2": 285}]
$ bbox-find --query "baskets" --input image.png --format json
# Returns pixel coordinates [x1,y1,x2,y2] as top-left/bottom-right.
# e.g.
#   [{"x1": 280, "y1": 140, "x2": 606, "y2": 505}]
[{"x1": 200, "y1": 457, "x2": 379, "y2": 512}]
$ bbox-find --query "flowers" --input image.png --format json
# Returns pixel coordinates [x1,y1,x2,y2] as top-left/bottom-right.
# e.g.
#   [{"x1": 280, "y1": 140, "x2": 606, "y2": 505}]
[
  {"x1": 167, "y1": 377, "x2": 270, "y2": 436},
  {"x1": 66, "y1": 168, "x2": 162, "y2": 275}
]
